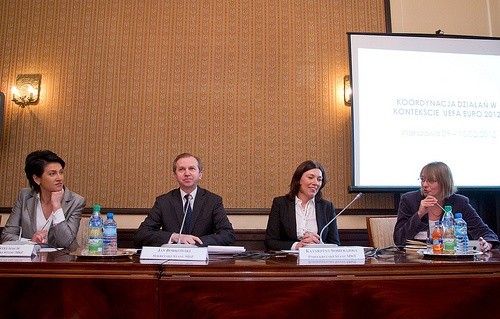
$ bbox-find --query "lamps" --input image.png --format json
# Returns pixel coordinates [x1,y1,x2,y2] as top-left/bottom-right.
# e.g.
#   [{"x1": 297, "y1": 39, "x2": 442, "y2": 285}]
[
  {"x1": 343, "y1": 75, "x2": 352, "y2": 106},
  {"x1": 11, "y1": 73, "x2": 42, "y2": 107}
]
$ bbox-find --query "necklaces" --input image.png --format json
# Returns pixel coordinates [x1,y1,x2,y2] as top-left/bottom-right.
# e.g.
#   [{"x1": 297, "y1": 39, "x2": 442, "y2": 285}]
[{"x1": 430, "y1": 207, "x2": 441, "y2": 217}]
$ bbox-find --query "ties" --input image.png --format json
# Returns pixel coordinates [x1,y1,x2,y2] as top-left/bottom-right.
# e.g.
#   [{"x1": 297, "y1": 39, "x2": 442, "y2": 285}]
[{"x1": 183, "y1": 194, "x2": 193, "y2": 233}]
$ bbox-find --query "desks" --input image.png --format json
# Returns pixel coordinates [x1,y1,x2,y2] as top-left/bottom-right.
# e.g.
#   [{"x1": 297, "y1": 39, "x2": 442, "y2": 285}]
[{"x1": 0, "y1": 251, "x2": 500, "y2": 319}]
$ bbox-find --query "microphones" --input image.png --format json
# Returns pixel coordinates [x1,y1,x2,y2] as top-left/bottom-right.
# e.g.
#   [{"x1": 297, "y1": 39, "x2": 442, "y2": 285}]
[
  {"x1": 303, "y1": 192, "x2": 363, "y2": 248},
  {"x1": 1, "y1": 225, "x2": 35, "y2": 246},
  {"x1": 161, "y1": 196, "x2": 199, "y2": 248}
]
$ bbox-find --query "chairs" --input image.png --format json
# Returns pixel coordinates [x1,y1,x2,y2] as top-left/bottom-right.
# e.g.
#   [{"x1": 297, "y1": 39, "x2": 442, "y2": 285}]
[{"x1": 365, "y1": 216, "x2": 398, "y2": 248}]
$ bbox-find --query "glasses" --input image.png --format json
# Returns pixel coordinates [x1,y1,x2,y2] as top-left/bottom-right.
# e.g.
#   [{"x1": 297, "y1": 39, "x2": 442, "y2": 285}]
[{"x1": 417, "y1": 177, "x2": 438, "y2": 183}]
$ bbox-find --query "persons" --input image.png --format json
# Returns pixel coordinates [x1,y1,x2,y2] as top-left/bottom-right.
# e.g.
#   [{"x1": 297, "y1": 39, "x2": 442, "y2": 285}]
[
  {"x1": 134, "y1": 153, "x2": 237, "y2": 247},
  {"x1": 1, "y1": 149, "x2": 86, "y2": 247},
  {"x1": 394, "y1": 162, "x2": 499, "y2": 253},
  {"x1": 264, "y1": 159, "x2": 340, "y2": 252}
]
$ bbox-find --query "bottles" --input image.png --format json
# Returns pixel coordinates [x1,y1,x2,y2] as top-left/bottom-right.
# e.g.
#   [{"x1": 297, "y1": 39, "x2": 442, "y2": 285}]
[
  {"x1": 453, "y1": 213, "x2": 469, "y2": 255},
  {"x1": 441, "y1": 205, "x2": 455, "y2": 254},
  {"x1": 81, "y1": 218, "x2": 90, "y2": 256},
  {"x1": 87, "y1": 205, "x2": 104, "y2": 256},
  {"x1": 432, "y1": 222, "x2": 443, "y2": 251},
  {"x1": 103, "y1": 213, "x2": 118, "y2": 256}
]
